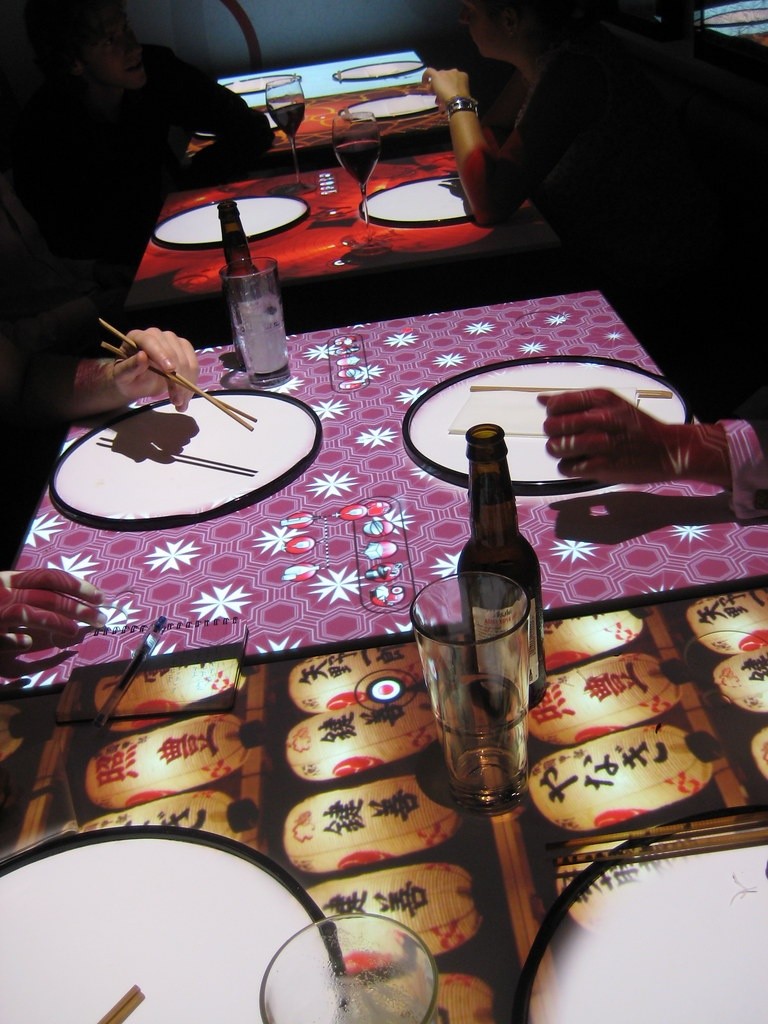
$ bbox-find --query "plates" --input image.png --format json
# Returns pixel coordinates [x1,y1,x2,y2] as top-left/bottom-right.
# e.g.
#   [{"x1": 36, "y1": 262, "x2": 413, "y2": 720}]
[
  {"x1": 331, "y1": 61, "x2": 425, "y2": 81},
  {"x1": 151, "y1": 196, "x2": 311, "y2": 249},
  {"x1": 195, "y1": 112, "x2": 278, "y2": 137},
  {"x1": 0, "y1": 827, "x2": 346, "y2": 1024},
  {"x1": 49, "y1": 389, "x2": 322, "y2": 531},
  {"x1": 359, "y1": 175, "x2": 472, "y2": 228},
  {"x1": 512, "y1": 804, "x2": 768, "y2": 1024},
  {"x1": 337, "y1": 94, "x2": 439, "y2": 120},
  {"x1": 403, "y1": 355, "x2": 690, "y2": 497},
  {"x1": 703, "y1": 8, "x2": 768, "y2": 26},
  {"x1": 222, "y1": 75, "x2": 301, "y2": 94}
]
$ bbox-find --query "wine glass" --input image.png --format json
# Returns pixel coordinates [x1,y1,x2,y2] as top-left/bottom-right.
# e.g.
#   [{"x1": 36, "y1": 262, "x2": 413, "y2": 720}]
[
  {"x1": 331, "y1": 112, "x2": 393, "y2": 256},
  {"x1": 265, "y1": 78, "x2": 318, "y2": 196}
]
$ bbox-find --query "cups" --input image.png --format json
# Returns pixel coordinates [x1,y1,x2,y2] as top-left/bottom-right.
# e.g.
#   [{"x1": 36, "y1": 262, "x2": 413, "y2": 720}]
[
  {"x1": 410, "y1": 571, "x2": 529, "y2": 817},
  {"x1": 258, "y1": 914, "x2": 439, "y2": 1024},
  {"x1": 218, "y1": 257, "x2": 291, "y2": 389}
]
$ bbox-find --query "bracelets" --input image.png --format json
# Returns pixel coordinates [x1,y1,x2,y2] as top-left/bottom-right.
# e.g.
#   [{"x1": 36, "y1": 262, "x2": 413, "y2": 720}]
[{"x1": 446, "y1": 94, "x2": 479, "y2": 120}]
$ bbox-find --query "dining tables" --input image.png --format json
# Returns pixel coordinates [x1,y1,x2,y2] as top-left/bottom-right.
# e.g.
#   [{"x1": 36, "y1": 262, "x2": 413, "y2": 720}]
[
  {"x1": 0, "y1": 571, "x2": 768, "y2": 1024},
  {"x1": 122, "y1": 149, "x2": 562, "y2": 313},
  {"x1": 0, "y1": 286, "x2": 768, "y2": 699},
  {"x1": 208, "y1": 47, "x2": 451, "y2": 160}
]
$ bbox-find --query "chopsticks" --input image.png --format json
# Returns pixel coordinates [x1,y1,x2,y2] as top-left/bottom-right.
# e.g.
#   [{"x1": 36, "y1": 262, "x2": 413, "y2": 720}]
[
  {"x1": 543, "y1": 811, "x2": 768, "y2": 865},
  {"x1": 97, "y1": 984, "x2": 145, "y2": 1024},
  {"x1": 98, "y1": 318, "x2": 257, "y2": 432},
  {"x1": 468, "y1": 385, "x2": 673, "y2": 399}
]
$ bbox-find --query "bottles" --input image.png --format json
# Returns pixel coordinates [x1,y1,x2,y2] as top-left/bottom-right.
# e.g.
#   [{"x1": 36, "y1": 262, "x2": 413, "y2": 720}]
[
  {"x1": 458, "y1": 423, "x2": 547, "y2": 711},
  {"x1": 216, "y1": 200, "x2": 262, "y2": 372}
]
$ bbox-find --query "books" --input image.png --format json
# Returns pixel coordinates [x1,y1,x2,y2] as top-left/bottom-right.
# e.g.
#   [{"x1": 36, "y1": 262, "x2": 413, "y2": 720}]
[{"x1": 53, "y1": 614, "x2": 252, "y2": 727}]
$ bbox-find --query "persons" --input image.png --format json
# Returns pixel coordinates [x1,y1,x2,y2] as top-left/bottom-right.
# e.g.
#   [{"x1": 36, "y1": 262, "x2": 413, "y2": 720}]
[
  {"x1": 0, "y1": 166, "x2": 237, "y2": 351},
  {"x1": 0, "y1": 325, "x2": 206, "y2": 697},
  {"x1": 11, "y1": 0, "x2": 275, "y2": 292},
  {"x1": 421, "y1": 0, "x2": 729, "y2": 349},
  {"x1": 534, "y1": 385, "x2": 768, "y2": 525}
]
